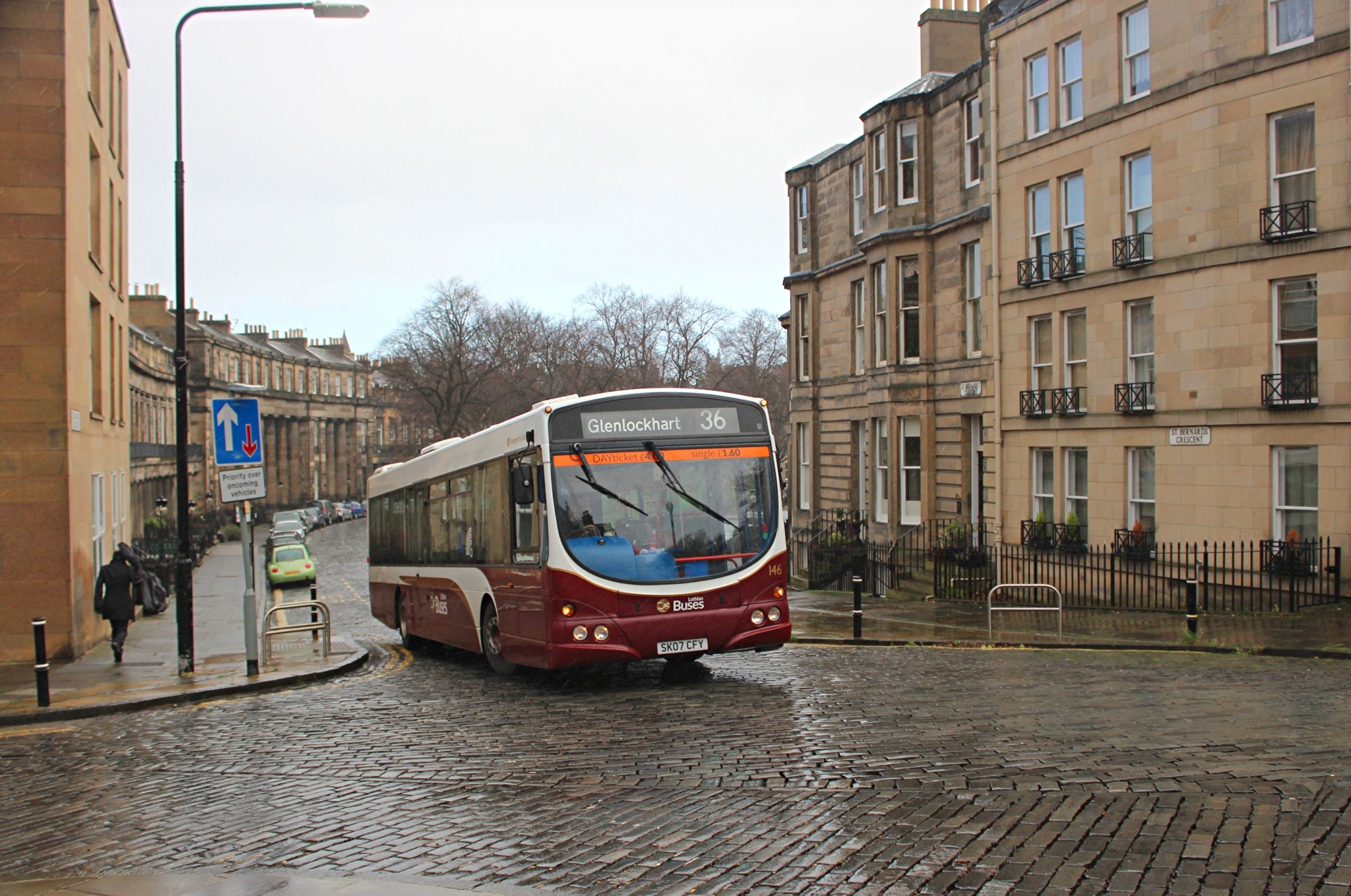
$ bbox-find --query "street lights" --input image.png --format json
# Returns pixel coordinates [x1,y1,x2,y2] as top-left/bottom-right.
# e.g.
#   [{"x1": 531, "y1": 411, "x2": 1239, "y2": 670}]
[
  {"x1": 226, "y1": 381, "x2": 266, "y2": 676},
  {"x1": 205, "y1": 492, "x2": 214, "y2": 547},
  {"x1": 188, "y1": 499, "x2": 197, "y2": 551},
  {"x1": 346, "y1": 478, "x2": 351, "y2": 501},
  {"x1": 155, "y1": 496, "x2": 167, "y2": 565},
  {"x1": 174, "y1": 0, "x2": 370, "y2": 683},
  {"x1": 278, "y1": 481, "x2": 284, "y2": 507}
]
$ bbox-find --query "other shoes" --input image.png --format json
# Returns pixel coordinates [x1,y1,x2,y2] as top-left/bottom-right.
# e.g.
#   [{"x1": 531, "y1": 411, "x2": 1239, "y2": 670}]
[
  {"x1": 111, "y1": 642, "x2": 121, "y2": 657},
  {"x1": 115, "y1": 656, "x2": 122, "y2": 661}
]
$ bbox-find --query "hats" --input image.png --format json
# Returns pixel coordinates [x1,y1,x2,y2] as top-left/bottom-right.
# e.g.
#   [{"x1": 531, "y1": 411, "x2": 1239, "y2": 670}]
[{"x1": 113, "y1": 550, "x2": 126, "y2": 561}]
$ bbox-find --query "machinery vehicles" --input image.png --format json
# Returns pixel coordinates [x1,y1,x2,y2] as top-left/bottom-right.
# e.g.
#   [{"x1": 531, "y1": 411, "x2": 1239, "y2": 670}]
[{"x1": 367, "y1": 386, "x2": 794, "y2": 677}]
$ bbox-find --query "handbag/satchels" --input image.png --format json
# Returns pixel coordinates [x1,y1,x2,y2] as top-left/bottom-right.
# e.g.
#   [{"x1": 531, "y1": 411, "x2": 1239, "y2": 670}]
[{"x1": 132, "y1": 583, "x2": 144, "y2": 605}]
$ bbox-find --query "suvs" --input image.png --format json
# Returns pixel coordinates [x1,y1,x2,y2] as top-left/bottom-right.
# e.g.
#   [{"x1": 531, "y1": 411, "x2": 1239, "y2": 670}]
[
  {"x1": 261, "y1": 531, "x2": 305, "y2": 557},
  {"x1": 305, "y1": 500, "x2": 339, "y2": 525}
]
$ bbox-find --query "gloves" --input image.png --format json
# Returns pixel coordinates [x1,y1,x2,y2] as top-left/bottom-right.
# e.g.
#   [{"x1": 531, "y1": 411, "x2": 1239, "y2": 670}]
[
  {"x1": 94, "y1": 603, "x2": 105, "y2": 615},
  {"x1": 127, "y1": 555, "x2": 139, "y2": 568}
]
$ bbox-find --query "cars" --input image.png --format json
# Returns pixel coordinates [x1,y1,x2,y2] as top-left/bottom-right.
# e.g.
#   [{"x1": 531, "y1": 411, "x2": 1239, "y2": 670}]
[
  {"x1": 343, "y1": 501, "x2": 367, "y2": 519},
  {"x1": 362, "y1": 500, "x2": 367, "y2": 509},
  {"x1": 265, "y1": 541, "x2": 313, "y2": 569},
  {"x1": 304, "y1": 507, "x2": 327, "y2": 528},
  {"x1": 269, "y1": 520, "x2": 308, "y2": 539},
  {"x1": 722, "y1": 509, "x2": 789, "y2": 554},
  {"x1": 270, "y1": 511, "x2": 309, "y2": 534},
  {"x1": 292, "y1": 510, "x2": 314, "y2": 530},
  {"x1": 267, "y1": 543, "x2": 316, "y2": 588},
  {"x1": 332, "y1": 503, "x2": 353, "y2": 521}
]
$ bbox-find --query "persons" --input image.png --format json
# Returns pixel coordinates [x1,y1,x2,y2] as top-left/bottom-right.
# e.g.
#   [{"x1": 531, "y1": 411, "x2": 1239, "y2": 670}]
[
  {"x1": 558, "y1": 498, "x2": 600, "y2": 539},
  {"x1": 93, "y1": 550, "x2": 144, "y2": 663}
]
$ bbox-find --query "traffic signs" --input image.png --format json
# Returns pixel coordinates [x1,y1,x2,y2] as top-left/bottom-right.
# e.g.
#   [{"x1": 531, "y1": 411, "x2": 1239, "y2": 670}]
[
  {"x1": 217, "y1": 466, "x2": 267, "y2": 505},
  {"x1": 211, "y1": 398, "x2": 263, "y2": 466}
]
